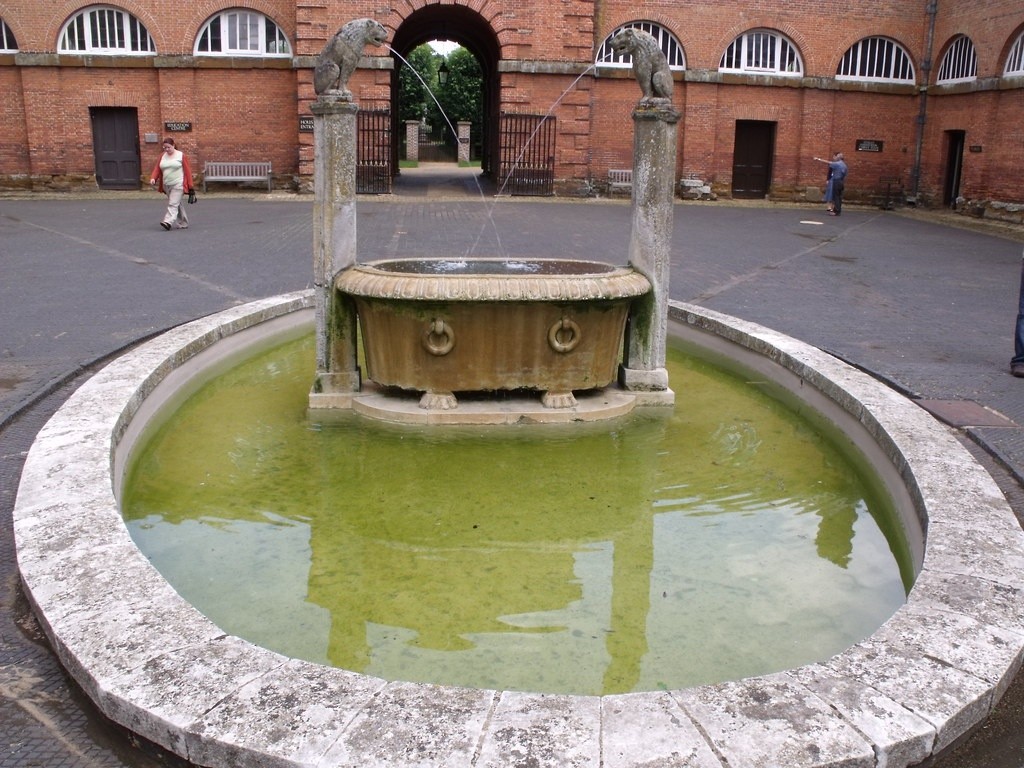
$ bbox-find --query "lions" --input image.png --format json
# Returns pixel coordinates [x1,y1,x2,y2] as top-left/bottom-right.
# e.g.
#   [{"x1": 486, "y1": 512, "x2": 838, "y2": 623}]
[
  {"x1": 608, "y1": 28, "x2": 673, "y2": 102},
  {"x1": 314, "y1": 18, "x2": 387, "y2": 96}
]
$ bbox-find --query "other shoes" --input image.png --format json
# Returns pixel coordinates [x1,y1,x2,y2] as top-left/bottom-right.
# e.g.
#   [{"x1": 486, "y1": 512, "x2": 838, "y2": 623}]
[
  {"x1": 160, "y1": 222, "x2": 171, "y2": 230},
  {"x1": 177, "y1": 225, "x2": 188, "y2": 229},
  {"x1": 827, "y1": 207, "x2": 840, "y2": 216}
]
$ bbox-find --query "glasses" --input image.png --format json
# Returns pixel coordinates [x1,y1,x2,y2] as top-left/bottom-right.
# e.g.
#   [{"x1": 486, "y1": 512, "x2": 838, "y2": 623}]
[{"x1": 163, "y1": 146, "x2": 171, "y2": 149}]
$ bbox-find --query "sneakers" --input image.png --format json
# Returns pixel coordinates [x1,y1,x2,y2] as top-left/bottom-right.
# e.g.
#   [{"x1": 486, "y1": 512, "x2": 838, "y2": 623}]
[{"x1": 1011, "y1": 365, "x2": 1024, "y2": 377}]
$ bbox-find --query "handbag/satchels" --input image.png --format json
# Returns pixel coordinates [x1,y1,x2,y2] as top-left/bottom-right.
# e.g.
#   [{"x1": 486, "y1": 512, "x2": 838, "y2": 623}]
[{"x1": 188, "y1": 189, "x2": 197, "y2": 204}]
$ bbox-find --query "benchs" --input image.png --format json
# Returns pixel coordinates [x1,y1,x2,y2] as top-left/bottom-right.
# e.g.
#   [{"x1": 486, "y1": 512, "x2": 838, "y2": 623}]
[
  {"x1": 608, "y1": 168, "x2": 632, "y2": 197},
  {"x1": 201, "y1": 160, "x2": 272, "y2": 192}
]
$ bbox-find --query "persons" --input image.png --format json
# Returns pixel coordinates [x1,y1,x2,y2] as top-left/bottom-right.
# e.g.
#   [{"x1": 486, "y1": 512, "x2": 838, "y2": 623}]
[
  {"x1": 813, "y1": 151, "x2": 849, "y2": 215},
  {"x1": 149, "y1": 137, "x2": 195, "y2": 231},
  {"x1": 1010, "y1": 246, "x2": 1024, "y2": 378},
  {"x1": 826, "y1": 153, "x2": 838, "y2": 212}
]
